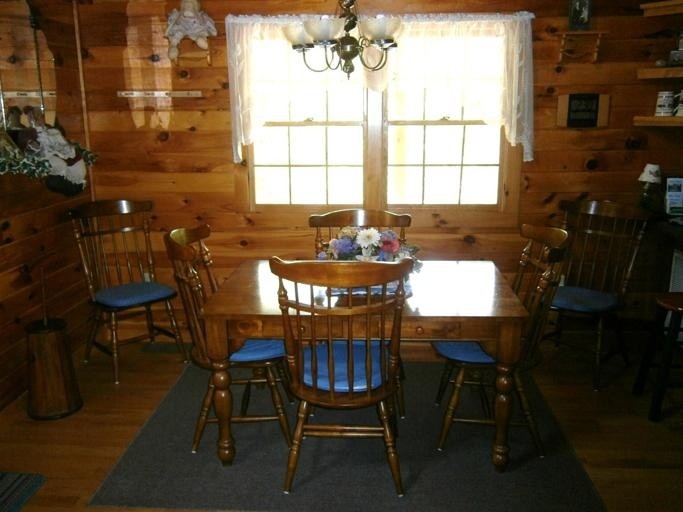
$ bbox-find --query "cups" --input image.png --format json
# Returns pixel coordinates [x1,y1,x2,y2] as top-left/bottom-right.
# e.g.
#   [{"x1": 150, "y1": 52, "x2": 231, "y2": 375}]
[{"x1": 654, "y1": 90, "x2": 675, "y2": 116}]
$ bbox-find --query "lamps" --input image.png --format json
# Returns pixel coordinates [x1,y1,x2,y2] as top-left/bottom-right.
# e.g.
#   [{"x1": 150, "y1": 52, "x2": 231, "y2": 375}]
[
  {"x1": 637, "y1": 162, "x2": 661, "y2": 208},
  {"x1": 280, "y1": 0, "x2": 406, "y2": 82}
]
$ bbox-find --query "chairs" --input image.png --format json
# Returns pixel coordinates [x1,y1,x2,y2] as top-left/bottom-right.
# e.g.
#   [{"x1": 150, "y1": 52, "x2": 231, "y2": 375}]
[
  {"x1": 430, "y1": 222, "x2": 572, "y2": 458},
  {"x1": 542, "y1": 198, "x2": 649, "y2": 391},
  {"x1": 65, "y1": 197, "x2": 188, "y2": 387},
  {"x1": 307, "y1": 207, "x2": 413, "y2": 244},
  {"x1": 265, "y1": 252, "x2": 418, "y2": 500},
  {"x1": 162, "y1": 222, "x2": 294, "y2": 455}
]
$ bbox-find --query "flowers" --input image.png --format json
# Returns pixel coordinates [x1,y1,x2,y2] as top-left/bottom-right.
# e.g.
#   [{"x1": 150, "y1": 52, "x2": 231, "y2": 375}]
[{"x1": 315, "y1": 225, "x2": 423, "y2": 282}]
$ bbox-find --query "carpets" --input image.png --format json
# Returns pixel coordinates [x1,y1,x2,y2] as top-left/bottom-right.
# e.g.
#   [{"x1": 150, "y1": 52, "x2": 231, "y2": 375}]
[
  {"x1": 86, "y1": 358, "x2": 612, "y2": 512},
  {"x1": 0, "y1": 470, "x2": 46, "y2": 512}
]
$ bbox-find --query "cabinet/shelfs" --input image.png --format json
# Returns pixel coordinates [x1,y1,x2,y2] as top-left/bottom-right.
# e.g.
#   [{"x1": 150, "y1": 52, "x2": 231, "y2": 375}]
[{"x1": 630, "y1": 0, "x2": 683, "y2": 128}]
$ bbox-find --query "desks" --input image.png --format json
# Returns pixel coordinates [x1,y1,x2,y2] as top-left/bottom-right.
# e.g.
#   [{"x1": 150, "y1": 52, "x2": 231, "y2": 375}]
[{"x1": 196, "y1": 258, "x2": 530, "y2": 472}]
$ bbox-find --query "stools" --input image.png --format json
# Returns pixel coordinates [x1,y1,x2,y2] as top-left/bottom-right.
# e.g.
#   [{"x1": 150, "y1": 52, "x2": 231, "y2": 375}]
[{"x1": 631, "y1": 292, "x2": 683, "y2": 422}]
